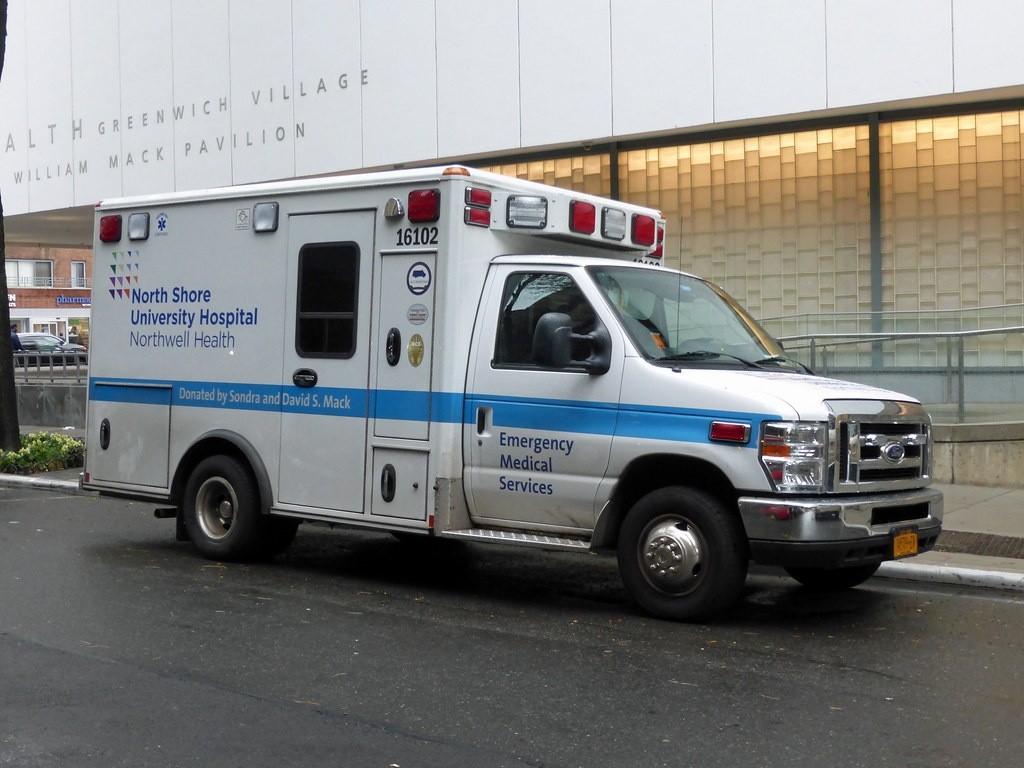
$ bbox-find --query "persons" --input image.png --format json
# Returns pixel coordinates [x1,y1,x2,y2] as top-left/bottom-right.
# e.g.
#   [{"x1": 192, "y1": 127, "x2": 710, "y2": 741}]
[
  {"x1": 11, "y1": 323, "x2": 25, "y2": 367},
  {"x1": 58, "y1": 333, "x2": 66, "y2": 353}
]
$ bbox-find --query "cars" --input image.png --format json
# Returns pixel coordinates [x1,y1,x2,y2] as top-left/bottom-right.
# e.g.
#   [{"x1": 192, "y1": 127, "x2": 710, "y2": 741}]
[{"x1": 15, "y1": 332, "x2": 87, "y2": 367}]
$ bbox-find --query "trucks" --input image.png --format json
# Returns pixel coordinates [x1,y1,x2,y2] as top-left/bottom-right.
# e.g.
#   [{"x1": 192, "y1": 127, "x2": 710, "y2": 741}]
[{"x1": 78, "y1": 164, "x2": 945, "y2": 622}]
